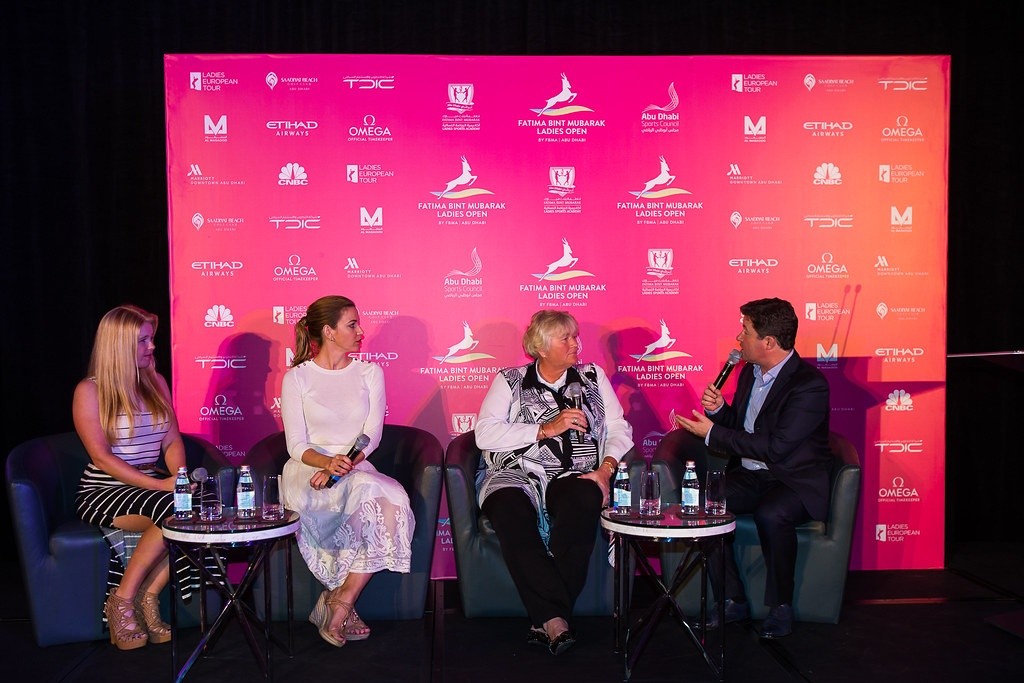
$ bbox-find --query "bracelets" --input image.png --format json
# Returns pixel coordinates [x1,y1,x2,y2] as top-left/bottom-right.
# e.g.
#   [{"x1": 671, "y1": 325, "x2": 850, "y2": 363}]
[
  {"x1": 540, "y1": 424, "x2": 549, "y2": 440},
  {"x1": 602, "y1": 461, "x2": 615, "y2": 474}
]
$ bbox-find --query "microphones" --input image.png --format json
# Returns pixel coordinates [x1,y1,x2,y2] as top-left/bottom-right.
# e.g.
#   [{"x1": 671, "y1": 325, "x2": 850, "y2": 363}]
[
  {"x1": 570, "y1": 382, "x2": 585, "y2": 444},
  {"x1": 325, "y1": 434, "x2": 371, "y2": 488},
  {"x1": 714, "y1": 348, "x2": 743, "y2": 391},
  {"x1": 188, "y1": 468, "x2": 208, "y2": 484}
]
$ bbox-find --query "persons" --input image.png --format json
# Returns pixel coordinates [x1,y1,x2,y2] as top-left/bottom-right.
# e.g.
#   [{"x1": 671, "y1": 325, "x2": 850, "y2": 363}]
[
  {"x1": 673, "y1": 296, "x2": 833, "y2": 640},
  {"x1": 72, "y1": 305, "x2": 230, "y2": 651},
  {"x1": 278, "y1": 294, "x2": 416, "y2": 649},
  {"x1": 474, "y1": 309, "x2": 636, "y2": 654}
]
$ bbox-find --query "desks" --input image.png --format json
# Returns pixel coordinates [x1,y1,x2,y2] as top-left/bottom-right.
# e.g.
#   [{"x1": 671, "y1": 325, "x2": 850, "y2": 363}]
[
  {"x1": 599, "y1": 502, "x2": 736, "y2": 683},
  {"x1": 162, "y1": 506, "x2": 304, "y2": 683}
]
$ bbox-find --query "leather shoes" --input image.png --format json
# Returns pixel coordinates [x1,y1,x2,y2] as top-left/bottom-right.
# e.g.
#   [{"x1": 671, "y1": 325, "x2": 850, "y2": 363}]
[
  {"x1": 760, "y1": 602, "x2": 794, "y2": 639},
  {"x1": 691, "y1": 598, "x2": 752, "y2": 631}
]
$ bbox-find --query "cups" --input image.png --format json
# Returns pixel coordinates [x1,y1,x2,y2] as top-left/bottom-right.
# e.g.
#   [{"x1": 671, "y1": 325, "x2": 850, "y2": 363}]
[
  {"x1": 705, "y1": 468, "x2": 728, "y2": 515},
  {"x1": 200, "y1": 476, "x2": 223, "y2": 521},
  {"x1": 639, "y1": 471, "x2": 661, "y2": 516},
  {"x1": 262, "y1": 474, "x2": 286, "y2": 520}
]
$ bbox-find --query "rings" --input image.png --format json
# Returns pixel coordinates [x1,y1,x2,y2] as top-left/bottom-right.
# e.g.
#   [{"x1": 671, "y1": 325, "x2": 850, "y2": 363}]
[{"x1": 572, "y1": 417, "x2": 577, "y2": 423}]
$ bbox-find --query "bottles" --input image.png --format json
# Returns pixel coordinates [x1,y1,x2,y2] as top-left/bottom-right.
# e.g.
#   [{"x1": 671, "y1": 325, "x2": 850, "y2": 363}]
[
  {"x1": 613, "y1": 462, "x2": 632, "y2": 515},
  {"x1": 237, "y1": 466, "x2": 256, "y2": 519},
  {"x1": 681, "y1": 460, "x2": 699, "y2": 515},
  {"x1": 172, "y1": 466, "x2": 192, "y2": 521}
]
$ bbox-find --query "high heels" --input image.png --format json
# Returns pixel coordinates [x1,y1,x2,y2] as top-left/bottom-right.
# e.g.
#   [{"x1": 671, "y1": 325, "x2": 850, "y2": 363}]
[
  {"x1": 344, "y1": 606, "x2": 370, "y2": 641},
  {"x1": 133, "y1": 589, "x2": 172, "y2": 644},
  {"x1": 308, "y1": 587, "x2": 353, "y2": 648},
  {"x1": 104, "y1": 587, "x2": 148, "y2": 650}
]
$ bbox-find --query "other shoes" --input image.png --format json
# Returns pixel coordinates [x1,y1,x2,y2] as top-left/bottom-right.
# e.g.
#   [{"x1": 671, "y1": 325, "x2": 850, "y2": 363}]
[
  {"x1": 549, "y1": 630, "x2": 582, "y2": 656},
  {"x1": 526, "y1": 628, "x2": 550, "y2": 648}
]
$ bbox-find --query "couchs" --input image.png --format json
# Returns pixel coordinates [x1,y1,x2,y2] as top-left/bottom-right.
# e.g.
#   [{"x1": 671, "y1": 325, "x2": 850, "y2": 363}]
[
  {"x1": 6, "y1": 427, "x2": 234, "y2": 649},
  {"x1": 650, "y1": 421, "x2": 863, "y2": 627},
  {"x1": 445, "y1": 428, "x2": 647, "y2": 622},
  {"x1": 236, "y1": 423, "x2": 443, "y2": 623}
]
